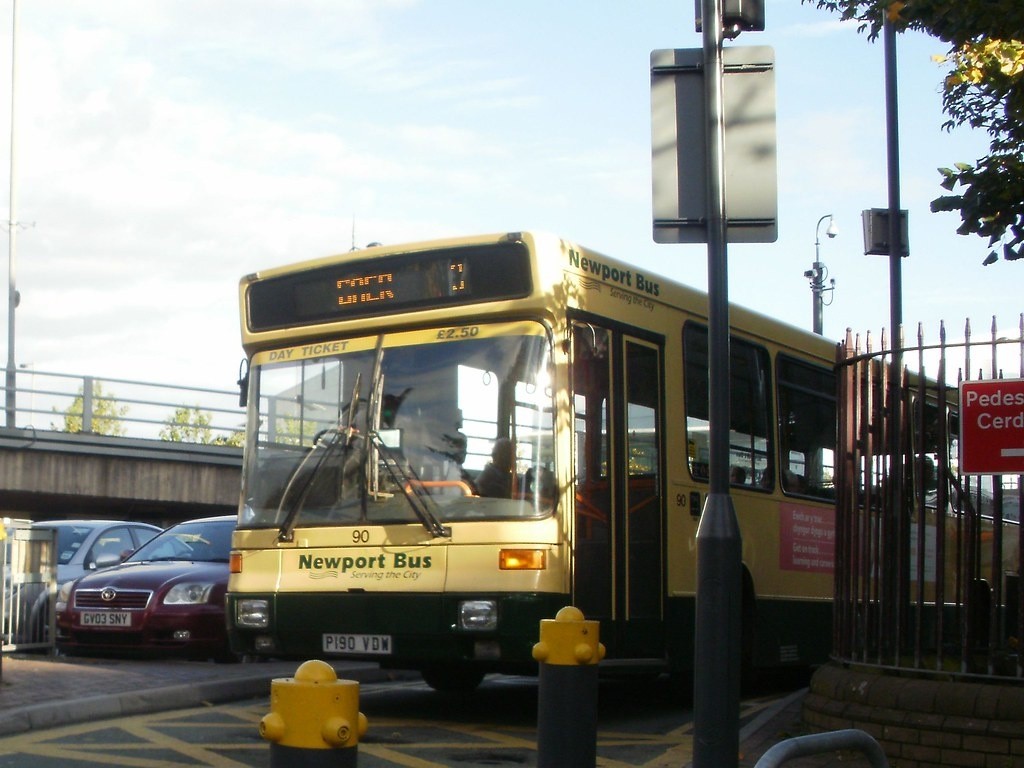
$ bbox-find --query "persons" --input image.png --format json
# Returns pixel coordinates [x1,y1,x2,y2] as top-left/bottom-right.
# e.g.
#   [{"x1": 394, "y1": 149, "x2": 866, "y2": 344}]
[
  {"x1": 759, "y1": 447, "x2": 805, "y2": 493},
  {"x1": 731, "y1": 467, "x2": 747, "y2": 483},
  {"x1": 474, "y1": 436, "x2": 520, "y2": 501},
  {"x1": 342, "y1": 410, "x2": 387, "y2": 490}
]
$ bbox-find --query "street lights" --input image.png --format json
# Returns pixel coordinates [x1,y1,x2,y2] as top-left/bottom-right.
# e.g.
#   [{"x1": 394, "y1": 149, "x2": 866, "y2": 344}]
[{"x1": 802, "y1": 212, "x2": 838, "y2": 334}]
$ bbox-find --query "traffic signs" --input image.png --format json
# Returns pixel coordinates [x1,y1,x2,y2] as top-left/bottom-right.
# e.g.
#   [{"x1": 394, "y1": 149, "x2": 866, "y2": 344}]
[{"x1": 955, "y1": 377, "x2": 1024, "y2": 478}]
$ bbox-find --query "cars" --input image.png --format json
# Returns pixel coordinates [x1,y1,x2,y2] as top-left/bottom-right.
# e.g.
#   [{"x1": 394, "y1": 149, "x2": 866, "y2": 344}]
[
  {"x1": 48, "y1": 513, "x2": 275, "y2": 663},
  {"x1": 0, "y1": 514, "x2": 195, "y2": 654}
]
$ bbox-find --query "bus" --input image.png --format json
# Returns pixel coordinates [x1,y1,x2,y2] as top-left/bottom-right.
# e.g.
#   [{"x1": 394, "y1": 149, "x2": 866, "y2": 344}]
[{"x1": 221, "y1": 228, "x2": 1024, "y2": 692}]
